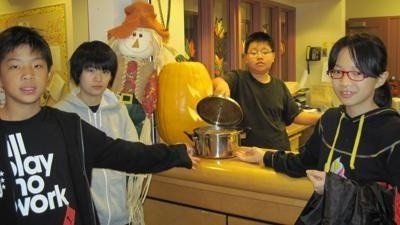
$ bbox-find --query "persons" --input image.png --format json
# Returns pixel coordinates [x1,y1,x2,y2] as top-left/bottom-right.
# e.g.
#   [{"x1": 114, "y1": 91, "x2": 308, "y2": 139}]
[
  {"x1": 0, "y1": 26, "x2": 201, "y2": 225},
  {"x1": 229, "y1": 30, "x2": 400, "y2": 225},
  {"x1": 48, "y1": 40, "x2": 140, "y2": 225},
  {"x1": 211, "y1": 31, "x2": 326, "y2": 152},
  {"x1": 106, "y1": 2, "x2": 170, "y2": 146}
]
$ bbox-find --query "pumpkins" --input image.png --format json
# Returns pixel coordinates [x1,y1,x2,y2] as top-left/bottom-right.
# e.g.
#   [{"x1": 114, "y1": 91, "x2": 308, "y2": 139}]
[{"x1": 156, "y1": 54, "x2": 214, "y2": 147}]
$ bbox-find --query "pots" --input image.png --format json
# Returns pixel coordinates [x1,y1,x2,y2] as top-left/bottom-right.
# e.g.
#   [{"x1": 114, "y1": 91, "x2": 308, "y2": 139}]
[{"x1": 184, "y1": 96, "x2": 252, "y2": 159}]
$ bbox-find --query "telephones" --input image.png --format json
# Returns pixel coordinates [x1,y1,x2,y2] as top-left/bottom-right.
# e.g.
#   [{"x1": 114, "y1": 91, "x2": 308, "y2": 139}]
[{"x1": 306, "y1": 46, "x2": 321, "y2": 61}]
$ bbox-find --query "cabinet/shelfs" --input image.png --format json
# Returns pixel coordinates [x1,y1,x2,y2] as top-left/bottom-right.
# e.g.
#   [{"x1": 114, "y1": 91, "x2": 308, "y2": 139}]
[{"x1": 125, "y1": 166, "x2": 317, "y2": 224}]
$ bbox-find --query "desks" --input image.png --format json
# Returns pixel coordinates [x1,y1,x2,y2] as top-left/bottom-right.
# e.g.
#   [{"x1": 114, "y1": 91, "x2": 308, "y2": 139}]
[{"x1": 284, "y1": 107, "x2": 324, "y2": 152}]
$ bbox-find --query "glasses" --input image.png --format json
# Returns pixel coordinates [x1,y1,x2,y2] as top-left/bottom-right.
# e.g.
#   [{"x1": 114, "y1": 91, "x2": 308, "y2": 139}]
[{"x1": 327, "y1": 69, "x2": 377, "y2": 81}]
